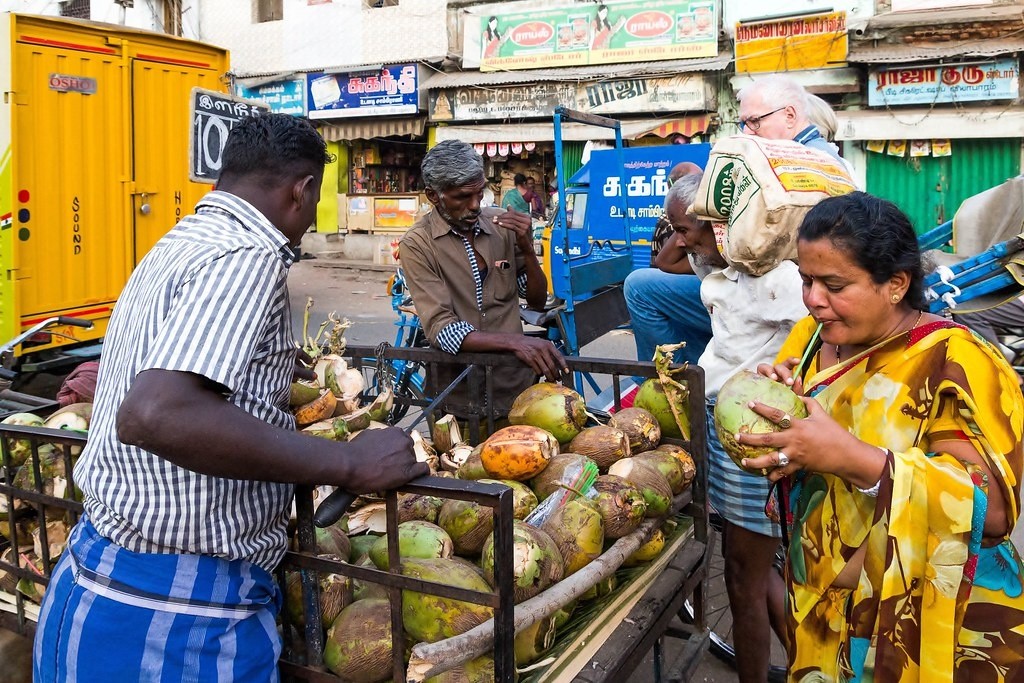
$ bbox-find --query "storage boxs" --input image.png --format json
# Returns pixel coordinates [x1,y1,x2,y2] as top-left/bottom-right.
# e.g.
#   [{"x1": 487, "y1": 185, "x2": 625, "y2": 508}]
[{"x1": 365, "y1": 149, "x2": 381, "y2": 164}]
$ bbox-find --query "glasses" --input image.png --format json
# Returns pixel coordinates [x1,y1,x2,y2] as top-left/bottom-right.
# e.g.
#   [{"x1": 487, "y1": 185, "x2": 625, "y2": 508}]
[{"x1": 736, "y1": 107, "x2": 786, "y2": 132}]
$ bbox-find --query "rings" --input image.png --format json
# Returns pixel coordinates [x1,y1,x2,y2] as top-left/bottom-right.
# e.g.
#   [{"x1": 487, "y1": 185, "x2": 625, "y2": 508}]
[
  {"x1": 778, "y1": 451, "x2": 789, "y2": 466},
  {"x1": 780, "y1": 413, "x2": 790, "y2": 428}
]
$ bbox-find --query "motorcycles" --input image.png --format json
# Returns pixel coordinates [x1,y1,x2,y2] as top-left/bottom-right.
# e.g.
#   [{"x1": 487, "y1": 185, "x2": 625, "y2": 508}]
[{"x1": 538, "y1": 141, "x2": 713, "y2": 312}]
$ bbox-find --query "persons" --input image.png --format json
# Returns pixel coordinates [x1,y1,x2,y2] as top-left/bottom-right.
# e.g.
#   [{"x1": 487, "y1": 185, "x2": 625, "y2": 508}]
[
  {"x1": 655, "y1": 172, "x2": 810, "y2": 681},
  {"x1": 649, "y1": 161, "x2": 703, "y2": 268},
  {"x1": 32, "y1": 114, "x2": 430, "y2": 683},
  {"x1": 808, "y1": 93, "x2": 856, "y2": 181},
  {"x1": 526, "y1": 177, "x2": 543, "y2": 218},
  {"x1": 481, "y1": 16, "x2": 513, "y2": 59},
  {"x1": 734, "y1": 190, "x2": 1024, "y2": 683},
  {"x1": 588, "y1": 5, "x2": 626, "y2": 51},
  {"x1": 624, "y1": 73, "x2": 850, "y2": 386},
  {"x1": 502, "y1": 173, "x2": 537, "y2": 215},
  {"x1": 398, "y1": 138, "x2": 570, "y2": 446}
]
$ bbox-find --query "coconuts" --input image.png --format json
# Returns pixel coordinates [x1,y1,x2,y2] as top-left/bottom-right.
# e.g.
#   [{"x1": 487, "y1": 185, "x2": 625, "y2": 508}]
[
  {"x1": 714, "y1": 369, "x2": 809, "y2": 475},
  {"x1": 277, "y1": 379, "x2": 697, "y2": 683},
  {"x1": 0, "y1": 401, "x2": 92, "y2": 603}
]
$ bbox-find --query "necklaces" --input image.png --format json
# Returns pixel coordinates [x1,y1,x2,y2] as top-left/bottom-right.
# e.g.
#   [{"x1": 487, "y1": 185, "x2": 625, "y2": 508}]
[{"x1": 836, "y1": 309, "x2": 924, "y2": 364}]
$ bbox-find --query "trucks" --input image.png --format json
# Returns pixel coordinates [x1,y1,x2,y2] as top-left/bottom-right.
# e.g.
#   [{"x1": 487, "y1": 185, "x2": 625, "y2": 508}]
[{"x1": 1, "y1": 10, "x2": 234, "y2": 373}]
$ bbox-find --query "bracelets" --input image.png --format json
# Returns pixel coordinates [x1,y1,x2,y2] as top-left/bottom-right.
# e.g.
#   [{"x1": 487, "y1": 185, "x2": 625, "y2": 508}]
[{"x1": 856, "y1": 446, "x2": 888, "y2": 497}]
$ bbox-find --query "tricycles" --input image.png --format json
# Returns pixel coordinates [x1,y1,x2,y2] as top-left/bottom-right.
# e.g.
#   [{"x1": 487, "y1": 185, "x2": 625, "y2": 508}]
[
  {"x1": 1, "y1": 316, "x2": 712, "y2": 681},
  {"x1": 329, "y1": 106, "x2": 1024, "y2": 441}
]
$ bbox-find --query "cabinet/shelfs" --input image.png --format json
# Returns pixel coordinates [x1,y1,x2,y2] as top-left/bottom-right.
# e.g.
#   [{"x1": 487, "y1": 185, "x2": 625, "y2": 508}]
[{"x1": 349, "y1": 141, "x2": 420, "y2": 193}]
